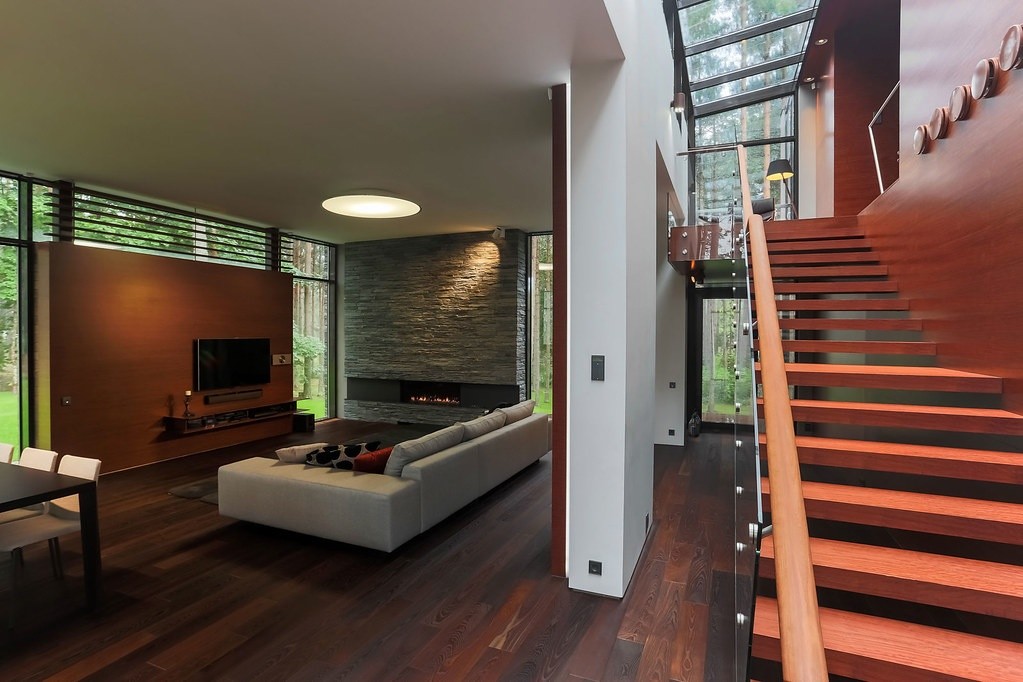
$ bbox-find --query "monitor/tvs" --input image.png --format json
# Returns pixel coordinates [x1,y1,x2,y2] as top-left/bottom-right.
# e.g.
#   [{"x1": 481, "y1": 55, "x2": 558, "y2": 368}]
[{"x1": 196, "y1": 338, "x2": 271, "y2": 392}]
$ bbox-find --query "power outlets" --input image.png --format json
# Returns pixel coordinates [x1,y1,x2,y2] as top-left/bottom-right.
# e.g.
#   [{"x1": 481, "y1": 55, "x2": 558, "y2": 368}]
[{"x1": 61, "y1": 396, "x2": 71, "y2": 406}]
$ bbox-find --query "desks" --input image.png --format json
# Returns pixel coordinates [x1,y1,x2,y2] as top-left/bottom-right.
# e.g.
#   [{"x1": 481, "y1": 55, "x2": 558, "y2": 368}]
[{"x1": 0, "y1": 462, "x2": 101, "y2": 602}]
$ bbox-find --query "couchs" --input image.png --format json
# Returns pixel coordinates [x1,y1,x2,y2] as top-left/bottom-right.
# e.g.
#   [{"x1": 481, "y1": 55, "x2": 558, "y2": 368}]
[{"x1": 217, "y1": 399, "x2": 553, "y2": 553}]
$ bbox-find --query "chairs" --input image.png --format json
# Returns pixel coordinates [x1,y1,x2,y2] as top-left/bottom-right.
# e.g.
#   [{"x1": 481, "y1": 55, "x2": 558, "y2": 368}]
[
  {"x1": 0, "y1": 443, "x2": 15, "y2": 568},
  {"x1": 751, "y1": 198, "x2": 774, "y2": 221},
  {"x1": 0, "y1": 447, "x2": 60, "y2": 581},
  {"x1": 0, "y1": 454, "x2": 101, "y2": 600}
]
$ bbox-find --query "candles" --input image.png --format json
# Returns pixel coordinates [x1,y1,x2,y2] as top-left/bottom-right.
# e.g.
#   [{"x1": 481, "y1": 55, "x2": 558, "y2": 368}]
[{"x1": 185, "y1": 391, "x2": 192, "y2": 396}]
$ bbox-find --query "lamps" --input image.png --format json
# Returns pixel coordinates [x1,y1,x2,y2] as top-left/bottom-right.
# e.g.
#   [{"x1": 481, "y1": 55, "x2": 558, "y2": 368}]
[
  {"x1": 765, "y1": 159, "x2": 798, "y2": 219},
  {"x1": 492, "y1": 226, "x2": 505, "y2": 239}
]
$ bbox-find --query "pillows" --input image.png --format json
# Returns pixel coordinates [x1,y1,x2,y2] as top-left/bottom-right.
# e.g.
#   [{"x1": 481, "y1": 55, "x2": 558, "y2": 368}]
[
  {"x1": 276, "y1": 442, "x2": 329, "y2": 463},
  {"x1": 330, "y1": 440, "x2": 381, "y2": 470},
  {"x1": 354, "y1": 445, "x2": 395, "y2": 475},
  {"x1": 305, "y1": 444, "x2": 352, "y2": 466},
  {"x1": 497, "y1": 399, "x2": 536, "y2": 426},
  {"x1": 459, "y1": 408, "x2": 507, "y2": 444},
  {"x1": 383, "y1": 421, "x2": 463, "y2": 477}
]
